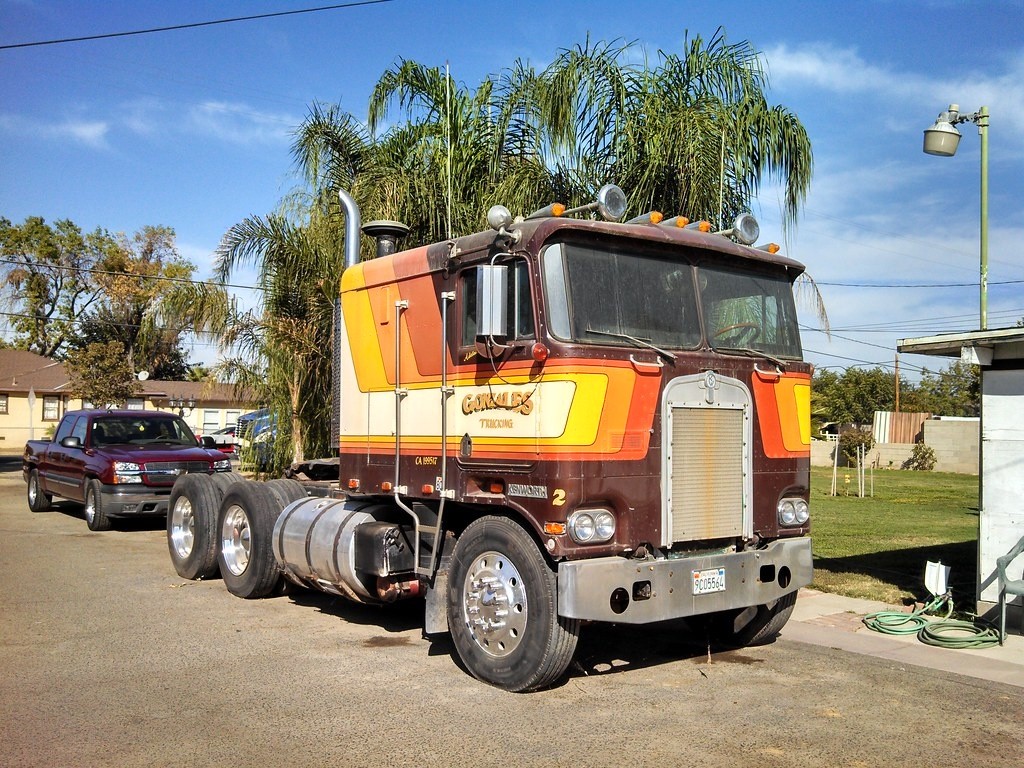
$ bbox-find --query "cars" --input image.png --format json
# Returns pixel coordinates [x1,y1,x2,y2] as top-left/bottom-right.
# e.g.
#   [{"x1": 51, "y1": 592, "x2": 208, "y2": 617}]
[{"x1": 200, "y1": 424, "x2": 238, "y2": 447}]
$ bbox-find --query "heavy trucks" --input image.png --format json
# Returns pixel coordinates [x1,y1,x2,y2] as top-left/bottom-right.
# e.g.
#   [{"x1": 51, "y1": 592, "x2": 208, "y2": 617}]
[{"x1": 167, "y1": 189, "x2": 812, "y2": 693}]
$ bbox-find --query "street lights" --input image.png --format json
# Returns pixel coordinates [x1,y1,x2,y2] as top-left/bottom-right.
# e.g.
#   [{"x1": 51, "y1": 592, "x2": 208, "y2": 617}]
[{"x1": 923, "y1": 103, "x2": 990, "y2": 333}]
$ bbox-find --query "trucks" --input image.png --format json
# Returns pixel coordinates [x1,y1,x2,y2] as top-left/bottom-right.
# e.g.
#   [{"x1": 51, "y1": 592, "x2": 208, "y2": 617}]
[{"x1": 233, "y1": 404, "x2": 282, "y2": 472}]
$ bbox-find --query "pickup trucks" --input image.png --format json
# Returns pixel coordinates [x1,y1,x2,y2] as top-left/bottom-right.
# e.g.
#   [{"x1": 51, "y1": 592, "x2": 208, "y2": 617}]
[{"x1": 22, "y1": 409, "x2": 231, "y2": 532}]
